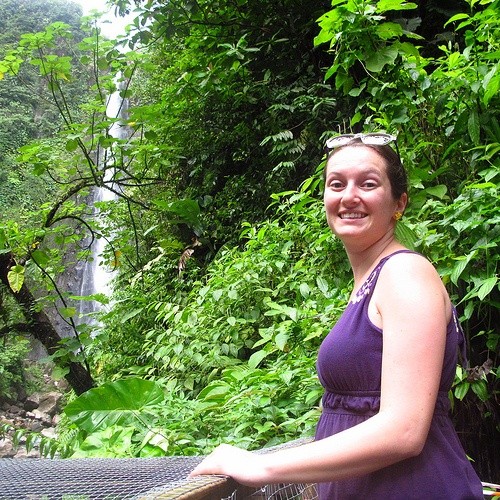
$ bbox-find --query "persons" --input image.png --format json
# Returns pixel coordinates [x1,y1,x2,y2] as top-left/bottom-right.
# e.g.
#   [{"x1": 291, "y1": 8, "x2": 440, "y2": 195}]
[{"x1": 183, "y1": 141, "x2": 483, "y2": 500}]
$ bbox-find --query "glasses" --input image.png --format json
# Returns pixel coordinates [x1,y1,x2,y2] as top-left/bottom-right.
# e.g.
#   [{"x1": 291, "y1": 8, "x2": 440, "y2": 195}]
[{"x1": 324, "y1": 131, "x2": 402, "y2": 168}]
